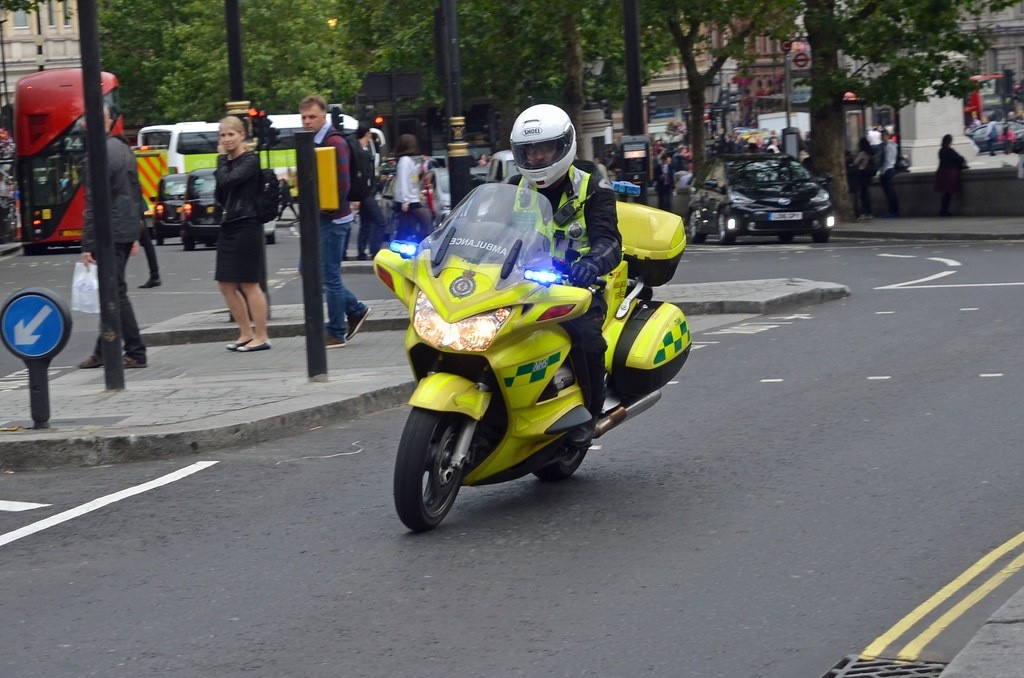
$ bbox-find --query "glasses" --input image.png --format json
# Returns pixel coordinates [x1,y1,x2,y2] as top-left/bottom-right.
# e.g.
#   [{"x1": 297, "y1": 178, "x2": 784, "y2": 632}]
[{"x1": 525, "y1": 141, "x2": 557, "y2": 155}]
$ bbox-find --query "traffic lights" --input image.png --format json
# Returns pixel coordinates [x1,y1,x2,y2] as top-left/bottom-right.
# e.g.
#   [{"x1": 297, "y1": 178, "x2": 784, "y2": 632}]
[
  {"x1": 492, "y1": 111, "x2": 502, "y2": 143},
  {"x1": 726, "y1": 87, "x2": 742, "y2": 111},
  {"x1": 646, "y1": 94, "x2": 658, "y2": 124},
  {"x1": 248, "y1": 108, "x2": 282, "y2": 152},
  {"x1": 371, "y1": 116, "x2": 384, "y2": 140},
  {"x1": 1001, "y1": 68, "x2": 1017, "y2": 99},
  {"x1": 482, "y1": 123, "x2": 491, "y2": 142},
  {"x1": 600, "y1": 98, "x2": 612, "y2": 119},
  {"x1": 332, "y1": 107, "x2": 344, "y2": 132}
]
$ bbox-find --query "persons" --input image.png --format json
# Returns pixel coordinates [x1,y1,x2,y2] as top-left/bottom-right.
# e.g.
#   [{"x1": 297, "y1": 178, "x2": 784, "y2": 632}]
[
  {"x1": 477, "y1": 104, "x2": 623, "y2": 448},
  {"x1": 80, "y1": 106, "x2": 162, "y2": 369},
  {"x1": 652, "y1": 131, "x2": 811, "y2": 197},
  {"x1": 874, "y1": 129, "x2": 900, "y2": 217},
  {"x1": 60, "y1": 172, "x2": 71, "y2": 199},
  {"x1": 867, "y1": 124, "x2": 888, "y2": 146},
  {"x1": 299, "y1": 95, "x2": 371, "y2": 348},
  {"x1": 848, "y1": 137, "x2": 875, "y2": 221},
  {"x1": 653, "y1": 156, "x2": 675, "y2": 211},
  {"x1": 276, "y1": 178, "x2": 299, "y2": 222},
  {"x1": 214, "y1": 116, "x2": 272, "y2": 352},
  {"x1": 342, "y1": 125, "x2": 433, "y2": 261},
  {"x1": 593, "y1": 154, "x2": 609, "y2": 180},
  {"x1": 478, "y1": 155, "x2": 493, "y2": 168},
  {"x1": 932, "y1": 134, "x2": 969, "y2": 215},
  {"x1": 965, "y1": 111, "x2": 1024, "y2": 156}
]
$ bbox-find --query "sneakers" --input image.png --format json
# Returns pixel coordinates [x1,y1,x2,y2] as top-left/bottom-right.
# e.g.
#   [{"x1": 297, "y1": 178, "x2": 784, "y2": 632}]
[
  {"x1": 121, "y1": 356, "x2": 147, "y2": 367},
  {"x1": 345, "y1": 307, "x2": 371, "y2": 341},
  {"x1": 326, "y1": 334, "x2": 346, "y2": 348},
  {"x1": 80, "y1": 353, "x2": 104, "y2": 368}
]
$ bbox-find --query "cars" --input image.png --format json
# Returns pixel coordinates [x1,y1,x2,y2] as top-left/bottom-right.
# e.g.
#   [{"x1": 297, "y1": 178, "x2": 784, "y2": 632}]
[
  {"x1": 148, "y1": 168, "x2": 280, "y2": 252},
  {"x1": 682, "y1": 153, "x2": 837, "y2": 246},
  {"x1": 963, "y1": 120, "x2": 1024, "y2": 154},
  {"x1": 379, "y1": 150, "x2": 520, "y2": 231}
]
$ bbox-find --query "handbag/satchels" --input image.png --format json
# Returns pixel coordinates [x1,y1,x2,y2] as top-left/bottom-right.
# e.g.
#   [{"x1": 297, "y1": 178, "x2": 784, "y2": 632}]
[
  {"x1": 70, "y1": 261, "x2": 101, "y2": 313},
  {"x1": 381, "y1": 174, "x2": 395, "y2": 199}
]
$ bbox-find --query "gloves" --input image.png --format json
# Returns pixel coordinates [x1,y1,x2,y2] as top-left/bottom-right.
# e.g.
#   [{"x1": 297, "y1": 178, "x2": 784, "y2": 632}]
[{"x1": 569, "y1": 258, "x2": 599, "y2": 289}]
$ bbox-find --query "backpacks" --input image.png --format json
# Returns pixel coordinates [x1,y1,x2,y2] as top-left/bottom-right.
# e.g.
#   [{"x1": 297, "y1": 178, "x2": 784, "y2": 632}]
[
  {"x1": 321, "y1": 128, "x2": 377, "y2": 202},
  {"x1": 235, "y1": 152, "x2": 279, "y2": 224}
]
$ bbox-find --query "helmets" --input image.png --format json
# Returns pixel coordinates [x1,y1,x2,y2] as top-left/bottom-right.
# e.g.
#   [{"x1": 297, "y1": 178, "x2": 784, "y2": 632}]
[{"x1": 510, "y1": 104, "x2": 577, "y2": 188}]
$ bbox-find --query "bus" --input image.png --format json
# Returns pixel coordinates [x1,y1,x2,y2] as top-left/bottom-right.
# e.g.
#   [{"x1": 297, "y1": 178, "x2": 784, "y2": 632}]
[
  {"x1": 137, "y1": 113, "x2": 388, "y2": 200},
  {"x1": 962, "y1": 72, "x2": 1021, "y2": 128},
  {"x1": 14, "y1": 67, "x2": 126, "y2": 254}
]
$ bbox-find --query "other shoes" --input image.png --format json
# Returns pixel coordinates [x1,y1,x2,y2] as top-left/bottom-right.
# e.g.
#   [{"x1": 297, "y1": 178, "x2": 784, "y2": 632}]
[
  {"x1": 225, "y1": 337, "x2": 255, "y2": 350},
  {"x1": 356, "y1": 254, "x2": 366, "y2": 260},
  {"x1": 341, "y1": 256, "x2": 348, "y2": 261},
  {"x1": 139, "y1": 277, "x2": 162, "y2": 288},
  {"x1": 237, "y1": 340, "x2": 271, "y2": 352},
  {"x1": 857, "y1": 214, "x2": 873, "y2": 220}
]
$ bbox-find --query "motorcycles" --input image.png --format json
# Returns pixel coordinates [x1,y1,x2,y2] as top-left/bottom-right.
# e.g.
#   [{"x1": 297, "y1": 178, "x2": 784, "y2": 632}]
[{"x1": 371, "y1": 181, "x2": 693, "y2": 533}]
